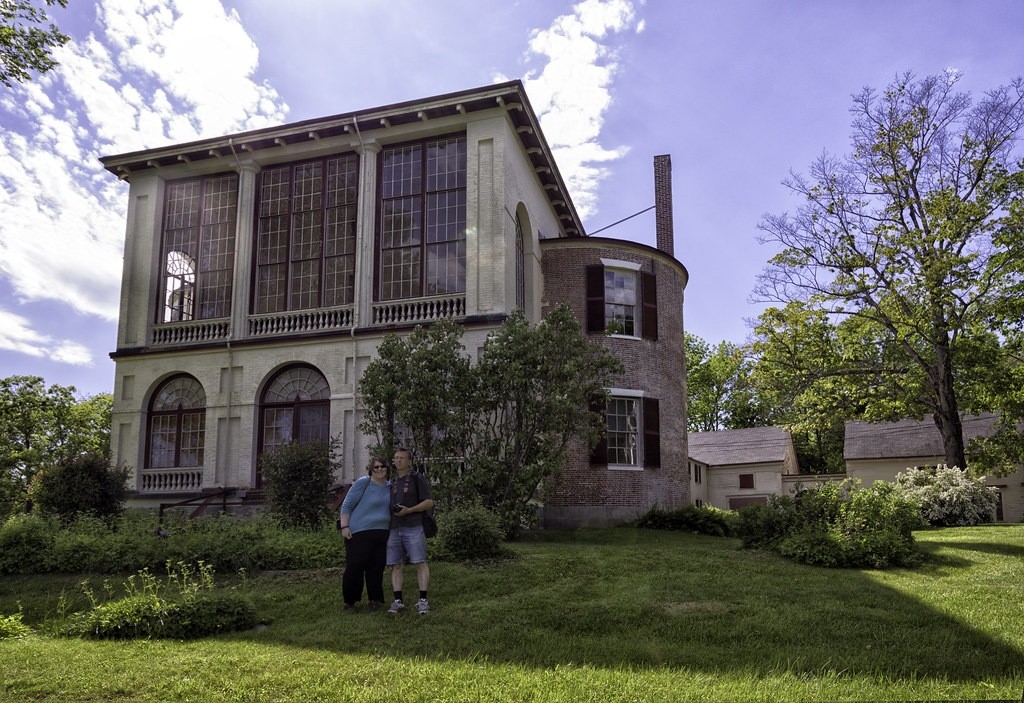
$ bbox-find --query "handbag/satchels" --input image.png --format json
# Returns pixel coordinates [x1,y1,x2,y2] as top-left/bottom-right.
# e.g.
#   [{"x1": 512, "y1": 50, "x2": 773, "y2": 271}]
[{"x1": 413, "y1": 472, "x2": 438, "y2": 539}]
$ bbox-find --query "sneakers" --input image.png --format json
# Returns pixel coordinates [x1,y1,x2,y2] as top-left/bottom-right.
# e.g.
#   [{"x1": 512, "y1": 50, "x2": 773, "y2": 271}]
[
  {"x1": 387, "y1": 599, "x2": 404, "y2": 612},
  {"x1": 415, "y1": 598, "x2": 430, "y2": 613}
]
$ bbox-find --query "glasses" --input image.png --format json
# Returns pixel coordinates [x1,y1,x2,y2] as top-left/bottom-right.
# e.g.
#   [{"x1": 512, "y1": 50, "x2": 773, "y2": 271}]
[{"x1": 374, "y1": 465, "x2": 385, "y2": 469}]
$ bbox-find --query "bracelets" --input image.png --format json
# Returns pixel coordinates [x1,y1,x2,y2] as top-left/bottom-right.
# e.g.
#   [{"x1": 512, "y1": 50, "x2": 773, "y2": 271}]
[{"x1": 341, "y1": 526, "x2": 348, "y2": 530}]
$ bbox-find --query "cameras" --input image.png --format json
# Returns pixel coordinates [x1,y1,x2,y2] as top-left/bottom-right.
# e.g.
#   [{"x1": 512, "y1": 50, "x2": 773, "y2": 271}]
[{"x1": 393, "y1": 503, "x2": 404, "y2": 513}]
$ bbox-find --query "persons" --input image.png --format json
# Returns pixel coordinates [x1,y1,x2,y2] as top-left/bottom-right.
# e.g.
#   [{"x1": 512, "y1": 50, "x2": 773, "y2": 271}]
[
  {"x1": 340, "y1": 458, "x2": 392, "y2": 612},
  {"x1": 386, "y1": 446, "x2": 434, "y2": 614}
]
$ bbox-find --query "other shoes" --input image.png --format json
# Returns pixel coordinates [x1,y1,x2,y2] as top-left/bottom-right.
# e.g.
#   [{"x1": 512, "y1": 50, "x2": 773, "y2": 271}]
[
  {"x1": 369, "y1": 600, "x2": 380, "y2": 612},
  {"x1": 342, "y1": 602, "x2": 357, "y2": 613}
]
